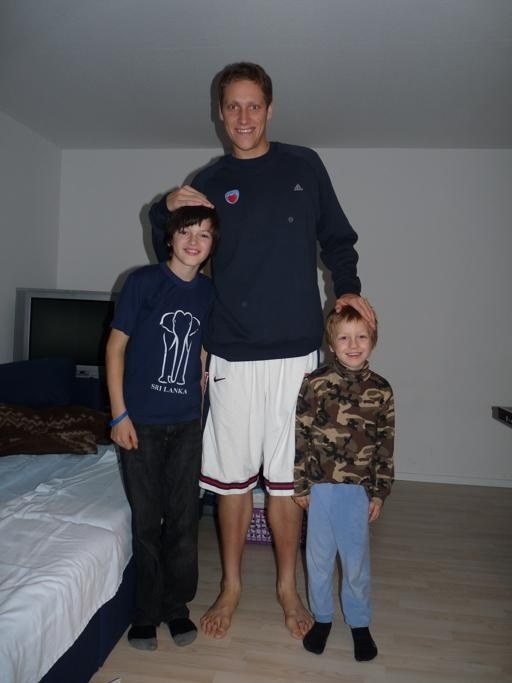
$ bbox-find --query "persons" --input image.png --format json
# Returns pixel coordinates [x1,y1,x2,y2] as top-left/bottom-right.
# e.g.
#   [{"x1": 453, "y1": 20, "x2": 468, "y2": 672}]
[
  {"x1": 104, "y1": 203, "x2": 216, "y2": 651},
  {"x1": 287, "y1": 306, "x2": 397, "y2": 661},
  {"x1": 146, "y1": 58, "x2": 378, "y2": 643}
]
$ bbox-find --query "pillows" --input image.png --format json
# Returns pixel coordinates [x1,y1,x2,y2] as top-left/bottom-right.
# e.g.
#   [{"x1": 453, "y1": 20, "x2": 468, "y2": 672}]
[{"x1": 0, "y1": 359, "x2": 115, "y2": 453}]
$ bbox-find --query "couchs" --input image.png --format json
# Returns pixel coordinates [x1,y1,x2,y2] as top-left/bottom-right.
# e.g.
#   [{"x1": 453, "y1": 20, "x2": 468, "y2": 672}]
[{"x1": 0, "y1": 354, "x2": 191, "y2": 683}]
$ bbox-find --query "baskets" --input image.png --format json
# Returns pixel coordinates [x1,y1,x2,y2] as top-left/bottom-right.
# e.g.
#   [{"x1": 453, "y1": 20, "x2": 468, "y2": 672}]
[{"x1": 243, "y1": 508, "x2": 275, "y2": 544}]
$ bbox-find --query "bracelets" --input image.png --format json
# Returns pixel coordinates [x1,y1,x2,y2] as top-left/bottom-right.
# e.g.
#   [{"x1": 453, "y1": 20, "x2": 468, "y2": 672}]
[{"x1": 109, "y1": 410, "x2": 130, "y2": 428}]
